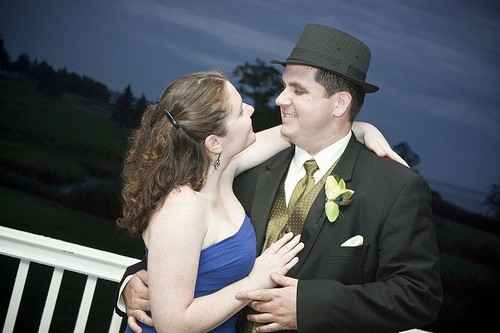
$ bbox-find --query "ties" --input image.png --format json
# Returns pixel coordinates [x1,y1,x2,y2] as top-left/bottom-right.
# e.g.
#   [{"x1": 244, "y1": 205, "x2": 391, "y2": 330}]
[{"x1": 282, "y1": 157, "x2": 320, "y2": 218}]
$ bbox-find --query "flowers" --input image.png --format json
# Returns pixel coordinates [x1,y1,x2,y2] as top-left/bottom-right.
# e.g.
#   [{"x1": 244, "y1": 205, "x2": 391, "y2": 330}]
[{"x1": 323, "y1": 171, "x2": 356, "y2": 223}]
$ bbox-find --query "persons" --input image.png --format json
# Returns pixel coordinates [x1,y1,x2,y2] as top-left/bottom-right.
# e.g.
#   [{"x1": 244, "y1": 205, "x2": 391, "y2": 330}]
[
  {"x1": 114, "y1": 24, "x2": 445, "y2": 333},
  {"x1": 113, "y1": 72, "x2": 412, "y2": 333}
]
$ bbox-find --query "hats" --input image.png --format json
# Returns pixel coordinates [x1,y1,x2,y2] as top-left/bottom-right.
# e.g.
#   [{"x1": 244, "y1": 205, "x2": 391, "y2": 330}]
[{"x1": 269, "y1": 23, "x2": 381, "y2": 94}]
